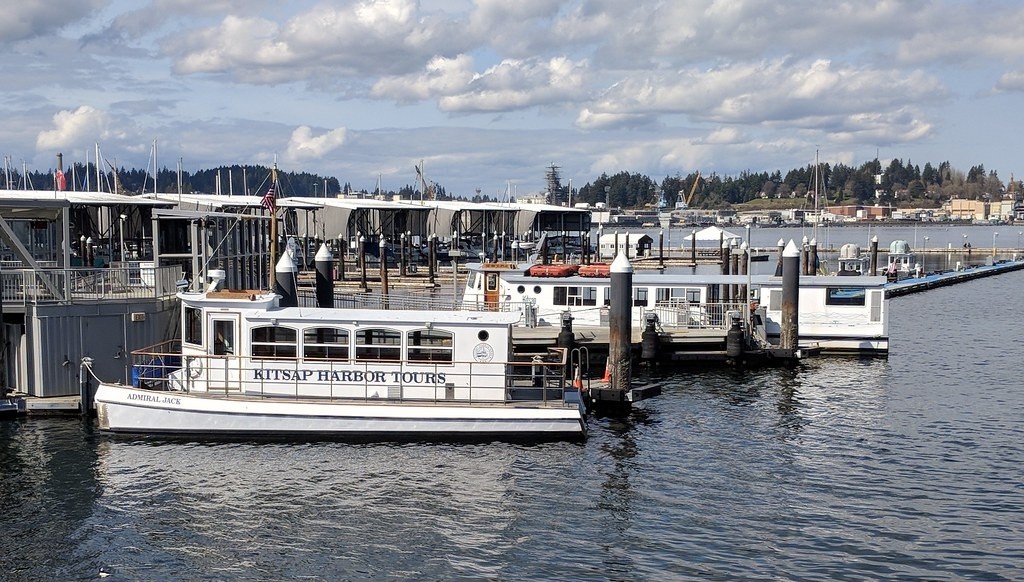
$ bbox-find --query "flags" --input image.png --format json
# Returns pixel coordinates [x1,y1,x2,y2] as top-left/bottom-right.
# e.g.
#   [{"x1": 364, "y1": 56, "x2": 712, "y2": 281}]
[
  {"x1": 56, "y1": 168, "x2": 66, "y2": 190},
  {"x1": 260, "y1": 183, "x2": 275, "y2": 213}
]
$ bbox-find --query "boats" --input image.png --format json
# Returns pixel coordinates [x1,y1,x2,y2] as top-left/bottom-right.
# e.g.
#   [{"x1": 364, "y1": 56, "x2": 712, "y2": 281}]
[
  {"x1": 0, "y1": 138, "x2": 595, "y2": 286},
  {"x1": 94, "y1": 154, "x2": 589, "y2": 446},
  {"x1": 459, "y1": 258, "x2": 891, "y2": 359}
]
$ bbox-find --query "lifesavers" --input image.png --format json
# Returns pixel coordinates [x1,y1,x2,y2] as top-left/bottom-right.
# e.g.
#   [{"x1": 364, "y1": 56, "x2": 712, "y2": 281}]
[{"x1": 183, "y1": 356, "x2": 203, "y2": 379}]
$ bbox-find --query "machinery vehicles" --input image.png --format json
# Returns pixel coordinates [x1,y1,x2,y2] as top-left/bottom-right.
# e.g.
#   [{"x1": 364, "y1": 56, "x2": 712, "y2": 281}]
[{"x1": 675, "y1": 171, "x2": 702, "y2": 209}]
